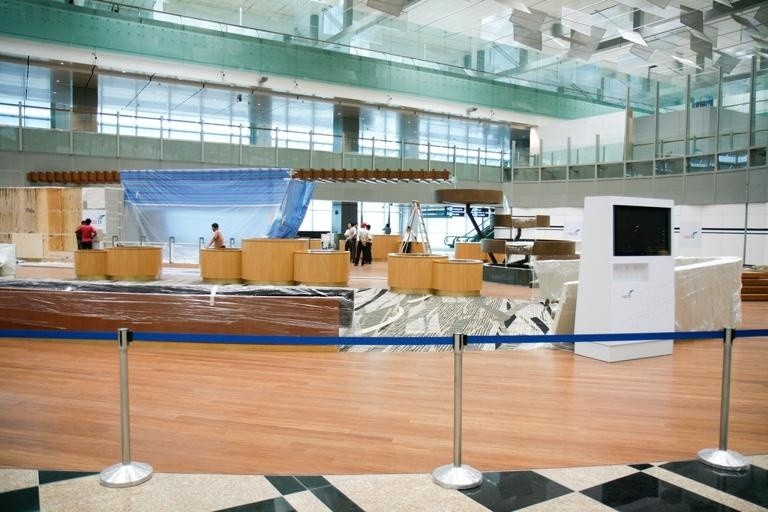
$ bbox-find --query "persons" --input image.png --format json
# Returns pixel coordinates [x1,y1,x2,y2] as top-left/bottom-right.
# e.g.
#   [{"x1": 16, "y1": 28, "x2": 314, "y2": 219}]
[
  {"x1": 73, "y1": 217, "x2": 96, "y2": 250},
  {"x1": 350, "y1": 222, "x2": 358, "y2": 259},
  {"x1": 205, "y1": 222, "x2": 226, "y2": 249},
  {"x1": 354, "y1": 223, "x2": 368, "y2": 267},
  {"x1": 75, "y1": 219, "x2": 85, "y2": 249},
  {"x1": 365, "y1": 224, "x2": 372, "y2": 264},
  {"x1": 382, "y1": 224, "x2": 391, "y2": 234},
  {"x1": 402, "y1": 226, "x2": 418, "y2": 253},
  {"x1": 344, "y1": 220, "x2": 354, "y2": 251}
]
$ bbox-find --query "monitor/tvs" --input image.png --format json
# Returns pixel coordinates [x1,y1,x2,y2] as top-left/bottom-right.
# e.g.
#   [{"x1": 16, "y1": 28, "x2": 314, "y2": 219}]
[{"x1": 613, "y1": 205, "x2": 672, "y2": 256}]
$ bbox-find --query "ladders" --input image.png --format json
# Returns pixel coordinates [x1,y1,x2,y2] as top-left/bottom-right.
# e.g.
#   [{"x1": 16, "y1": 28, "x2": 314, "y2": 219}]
[{"x1": 398, "y1": 200, "x2": 432, "y2": 254}]
